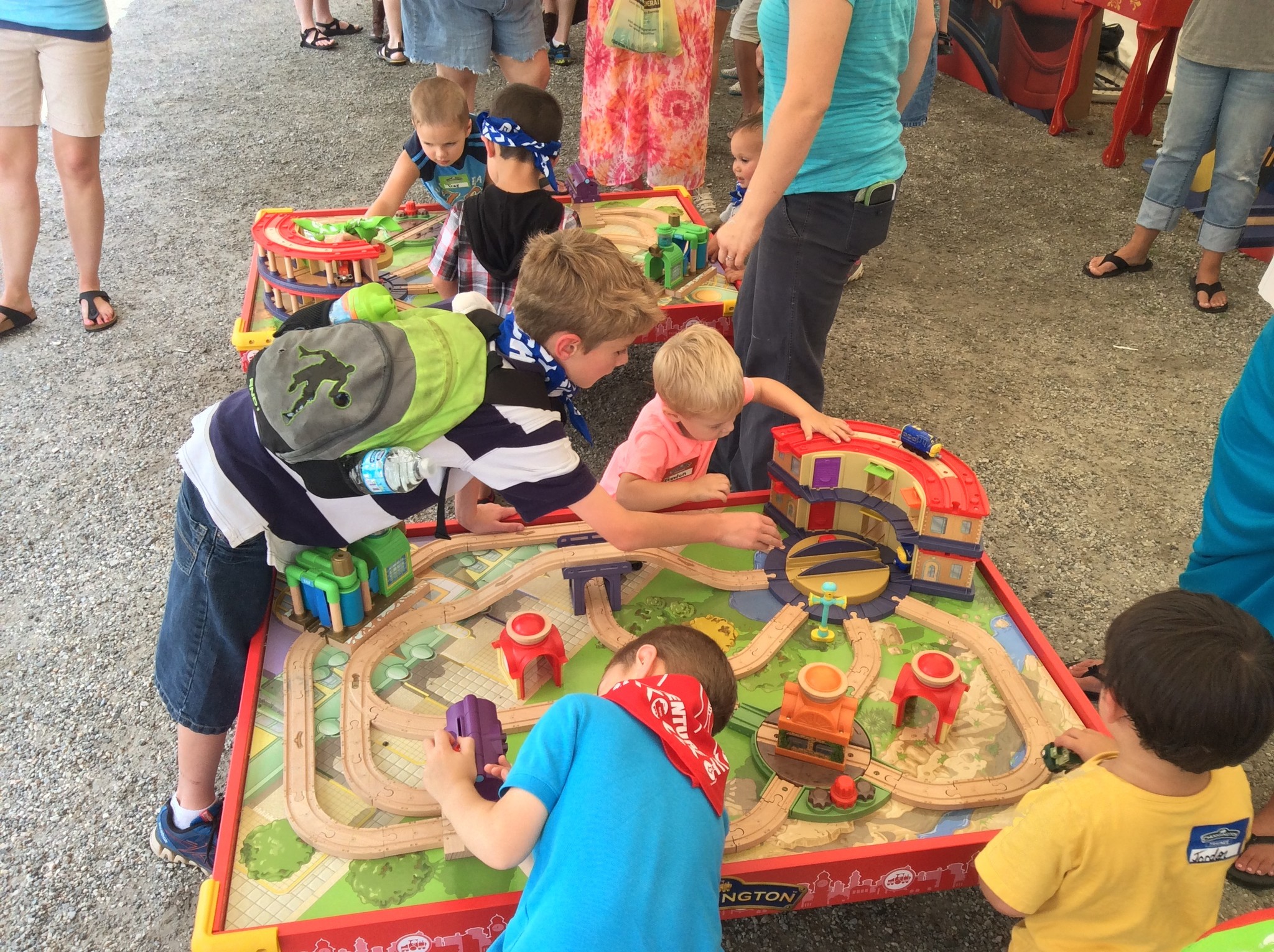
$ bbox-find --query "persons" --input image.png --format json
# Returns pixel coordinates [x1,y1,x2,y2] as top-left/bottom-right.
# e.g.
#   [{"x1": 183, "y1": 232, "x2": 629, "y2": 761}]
[
  {"x1": 0, "y1": 0, "x2": 116, "y2": 334},
  {"x1": 295, "y1": 1, "x2": 362, "y2": 49},
  {"x1": 1065, "y1": 237, "x2": 1274, "y2": 892},
  {"x1": 424, "y1": 625, "x2": 738, "y2": 952},
  {"x1": 326, "y1": 77, "x2": 491, "y2": 244},
  {"x1": 710, "y1": 0, "x2": 738, "y2": 106},
  {"x1": 709, "y1": 0, "x2": 936, "y2": 493},
  {"x1": 720, "y1": 109, "x2": 767, "y2": 284},
  {"x1": 147, "y1": 230, "x2": 787, "y2": 882},
  {"x1": 972, "y1": 591, "x2": 1274, "y2": 951},
  {"x1": 1083, "y1": 0, "x2": 1274, "y2": 313},
  {"x1": 400, "y1": 0, "x2": 551, "y2": 113},
  {"x1": 541, "y1": 65, "x2": 723, "y2": 232},
  {"x1": 728, "y1": 0, "x2": 764, "y2": 140},
  {"x1": 601, "y1": 320, "x2": 854, "y2": 512},
  {"x1": 375, "y1": 0, "x2": 408, "y2": 66},
  {"x1": 431, "y1": 82, "x2": 586, "y2": 321}
]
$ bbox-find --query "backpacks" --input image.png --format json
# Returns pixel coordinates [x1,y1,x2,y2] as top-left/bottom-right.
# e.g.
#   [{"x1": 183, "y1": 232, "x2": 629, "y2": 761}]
[{"x1": 240, "y1": 282, "x2": 568, "y2": 501}]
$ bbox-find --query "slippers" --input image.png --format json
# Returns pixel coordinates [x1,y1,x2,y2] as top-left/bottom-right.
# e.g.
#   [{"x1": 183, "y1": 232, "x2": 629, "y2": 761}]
[
  {"x1": 1083, "y1": 249, "x2": 1154, "y2": 278},
  {"x1": 1223, "y1": 829, "x2": 1274, "y2": 889},
  {"x1": 1062, "y1": 656, "x2": 1106, "y2": 701},
  {"x1": 78, "y1": 288, "x2": 117, "y2": 332},
  {"x1": 0, "y1": 304, "x2": 36, "y2": 336},
  {"x1": 1190, "y1": 275, "x2": 1229, "y2": 314}
]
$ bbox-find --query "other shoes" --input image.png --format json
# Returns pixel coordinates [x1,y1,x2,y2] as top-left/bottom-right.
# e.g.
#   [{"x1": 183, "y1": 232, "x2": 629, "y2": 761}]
[
  {"x1": 720, "y1": 65, "x2": 738, "y2": 79},
  {"x1": 369, "y1": 27, "x2": 385, "y2": 42},
  {"x1": 842, "y1": 256, "x2": 864, "y2": 286},
  {"x1": 727, "y1": 77, "x2": 764, "y2": 95}
]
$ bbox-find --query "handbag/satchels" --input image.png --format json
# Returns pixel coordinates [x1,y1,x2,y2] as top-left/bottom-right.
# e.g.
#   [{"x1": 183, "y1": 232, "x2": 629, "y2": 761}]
[{"x1": 600, "y1": 0, "x2": 682, "y2": 59}]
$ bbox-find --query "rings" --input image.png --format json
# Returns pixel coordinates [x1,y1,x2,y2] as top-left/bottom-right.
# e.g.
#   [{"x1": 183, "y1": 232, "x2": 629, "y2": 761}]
[{"x1": 725, "y1": 256, "x2": 734, "y2": 261}]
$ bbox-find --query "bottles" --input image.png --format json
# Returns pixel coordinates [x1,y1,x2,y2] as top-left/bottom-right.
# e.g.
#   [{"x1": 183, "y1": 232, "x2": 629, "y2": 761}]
[{"x1": 349, "y1": 446, "x2": 436, "y2": 495}]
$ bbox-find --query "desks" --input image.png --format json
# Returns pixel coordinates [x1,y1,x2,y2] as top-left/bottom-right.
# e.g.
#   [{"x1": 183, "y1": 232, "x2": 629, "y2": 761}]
[
  {"x1": 231, "y1": 183, "x2": 748, "y2": 377},
  {"x1": 1041, "y1": 0, "x2": 1200, "y2": 168},
  {"x1": 188, "y1": 473, "x2": 1120, "y2": 952}
]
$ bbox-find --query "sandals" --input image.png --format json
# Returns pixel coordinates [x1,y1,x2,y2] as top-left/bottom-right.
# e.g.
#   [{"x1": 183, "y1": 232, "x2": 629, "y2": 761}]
[
  {"x1": 316, "y1": 18, "x2": 364, "y2": 37},
  {"x1": 299, "y1": 28, "x2": 338, "y2": 50},
  {"x1": 612, "y1": 184, "x2": 642, "y2": 193},
  {"x1": 937, "y1": 31, "x2": 953, "y2": 55},
  {"x1": 374, "y1": 41, "x2": 407, "y2": 66},
  {"x1": 726, "y1": 101, "x2": 764, "y2": 139},
  {"x1": 687, "y1": 181, "x2": 717, "y2": 216}
]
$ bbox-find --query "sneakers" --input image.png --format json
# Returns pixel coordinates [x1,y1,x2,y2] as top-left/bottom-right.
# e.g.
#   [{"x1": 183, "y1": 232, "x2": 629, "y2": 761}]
[{"x1": 545, "y1": 38, "x2": 571, "y2": 65}]
